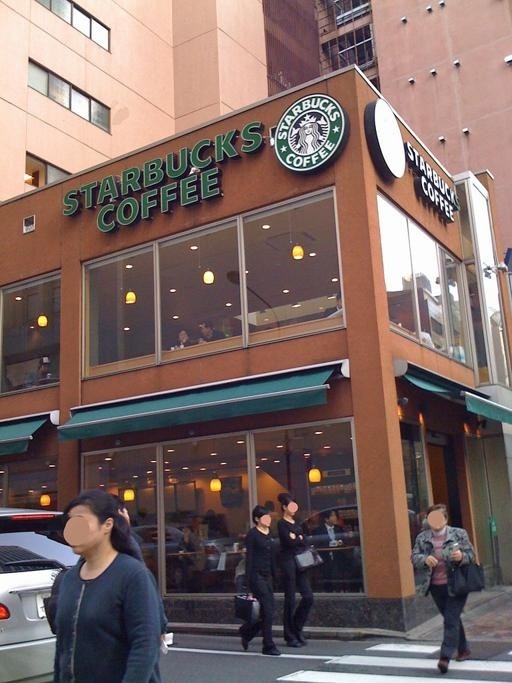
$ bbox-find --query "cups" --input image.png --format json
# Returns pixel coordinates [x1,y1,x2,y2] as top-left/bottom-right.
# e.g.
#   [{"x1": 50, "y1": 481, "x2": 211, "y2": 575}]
[{"x1": 233, "y1": 542, "x2": 240, "y2": 552}]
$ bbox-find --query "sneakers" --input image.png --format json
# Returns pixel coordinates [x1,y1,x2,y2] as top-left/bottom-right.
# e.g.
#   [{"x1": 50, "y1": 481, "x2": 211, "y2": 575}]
[
  {"x1": 438, "y1": 659, "x2": 448, "y2": 672},
  {"x1": 456, "y1": 648, "x2": 472, "y2": 660}
]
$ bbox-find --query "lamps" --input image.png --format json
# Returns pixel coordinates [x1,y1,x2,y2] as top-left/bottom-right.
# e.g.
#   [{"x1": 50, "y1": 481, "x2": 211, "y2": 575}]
[
  {"x1": 203, "y1": 268, "x2": 214, "y2": 285},
  {"x1": 309, "y1": 464, "x2": 322, "y2": 482},
  {"x1": 484, "y1": 261, "x2": 509, "y2": 272},
  {"x1": 40, "y1": 494, "x2": 51, "y2": 506},
  {"x1": 37, "y1": 313, "x2": 48, "y2": 327},
  {"x1": 210, "y1": 475, "x2": 222, "y2": 492},
  {"x1": 292, "y1": 243, "x2": 304, "y2": 260},
  {"x1": 477, "y1": 420, "x2": 486, "y2": 429},
  {"x1": 124, "y1": 489, "x2": 135, "y2": 502},
  {"x1": 126, "y1": 292, "x2": 137, "y2": 304}
]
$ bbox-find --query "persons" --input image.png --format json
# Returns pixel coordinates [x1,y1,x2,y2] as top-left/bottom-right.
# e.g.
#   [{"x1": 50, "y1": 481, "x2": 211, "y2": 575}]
[
  {"x1": 33, "y1": 356, "x2": 52, "y2": 386},
  {"x1": 238, "y1": 505, "x2": 282, "y2": 656},
  {"x1": 320, "y1": 291, "x2": 342, "y2": 319},
  {"x1": 408, "y1": 509, "x2": 428, "y2": 576},
  {"x1": 192, "y1": 319, "x2": 225, "y2": 343},
  {"x1": 167, "y1": 329, "x2": 191, "y2": 349},
  {"x1": 76, "y1": 493, "x2": 169, "y2": 646},
  {"x1": 138, "y1": 502, "x2": 347, "y2": 589},
  {"x1": 52, "y1": 487, "x2": 161, "y2": 683},
  {"x1": 410, "y1": 502, "x2": 476, "y2": 674},
  {"x1": 391, "y1": 319, "x2": 402, "y2": 327},
  {"x1": 276, "y1": 492, "x2": 315, "y2": 648}
]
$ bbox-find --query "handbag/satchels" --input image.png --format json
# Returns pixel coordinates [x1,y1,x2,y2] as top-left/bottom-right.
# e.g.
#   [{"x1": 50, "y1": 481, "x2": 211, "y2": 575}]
[
  {"x1": 295, "y1": 549, "x2": 324, "y2": 572},
  {"x1": 448, "y1": 562, "x2": 485, "y2": 596},
  {"x1": 234, "y1": 595, "x2": 261, "y2": 627}
]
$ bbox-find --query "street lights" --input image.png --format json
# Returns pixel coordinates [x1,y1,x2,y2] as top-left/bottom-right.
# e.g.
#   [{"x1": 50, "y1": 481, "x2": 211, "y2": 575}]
[{"x1": 225, "y1": 266, "x2": 292, "y2": 523}]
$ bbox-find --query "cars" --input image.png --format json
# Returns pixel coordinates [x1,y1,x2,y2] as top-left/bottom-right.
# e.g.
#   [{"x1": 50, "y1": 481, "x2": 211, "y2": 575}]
[{"x1": 0, "y1": 505, "x2": 159, "y2": 683}]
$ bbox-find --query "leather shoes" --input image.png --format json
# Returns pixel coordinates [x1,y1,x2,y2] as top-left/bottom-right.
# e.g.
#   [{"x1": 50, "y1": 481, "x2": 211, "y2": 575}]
[
  {"x1": 287, "y1": 627, "x2": 306, "y2": 648},
  {"x1": 239, "y1": 626, "x2": 249, "y2": 650},
  {"x1": 262, "y1": 645, "x2": 281, "y2": 655}
]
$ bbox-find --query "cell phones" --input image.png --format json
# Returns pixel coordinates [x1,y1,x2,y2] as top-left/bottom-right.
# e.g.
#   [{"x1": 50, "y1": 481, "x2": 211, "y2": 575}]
[{"x1": 453, "y1": 543, "x2": 459, "y2": 552}]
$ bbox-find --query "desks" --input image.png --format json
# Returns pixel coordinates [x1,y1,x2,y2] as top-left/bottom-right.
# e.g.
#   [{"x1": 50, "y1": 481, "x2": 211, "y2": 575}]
[
  {"x1": 318, "y1": 546, "x2": 355, "y2": 560},
  {"x1": 167, "y1": 552, "x2": 196, "y2": 573}
]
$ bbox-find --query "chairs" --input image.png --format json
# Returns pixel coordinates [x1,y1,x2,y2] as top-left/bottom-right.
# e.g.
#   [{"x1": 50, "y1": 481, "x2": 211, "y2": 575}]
[{"x1": 200, "y1": 553, "x2": 242, "y2": 593}]
